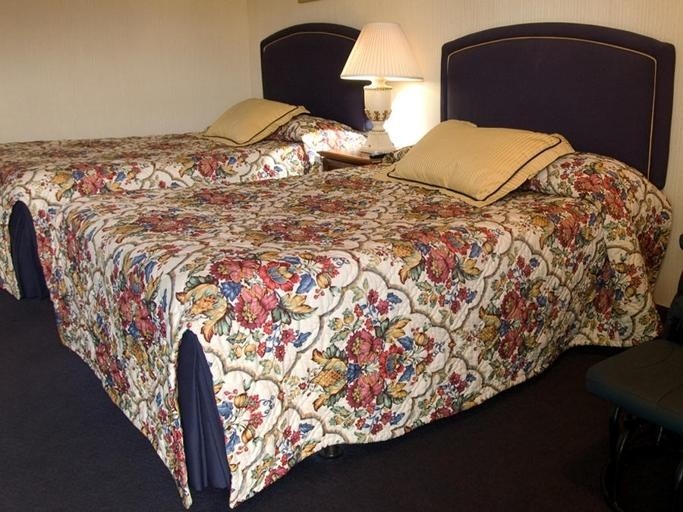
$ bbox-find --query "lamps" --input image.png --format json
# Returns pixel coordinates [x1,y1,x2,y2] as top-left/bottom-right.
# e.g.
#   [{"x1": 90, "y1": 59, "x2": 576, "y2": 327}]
[{"x1": 339, "y1": 21, "x2": 425, "y2": 155}]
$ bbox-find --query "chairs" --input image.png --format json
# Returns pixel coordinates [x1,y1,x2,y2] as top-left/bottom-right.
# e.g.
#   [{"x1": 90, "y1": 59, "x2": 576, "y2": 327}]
[{"x1": 586, "y1": 235, "x2": 681, "y2": 510}]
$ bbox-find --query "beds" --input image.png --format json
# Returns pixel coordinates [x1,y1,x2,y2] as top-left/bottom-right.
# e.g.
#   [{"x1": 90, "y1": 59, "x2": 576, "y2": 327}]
[
  {"x1": 2, "y1": 22, "x2": 371, "y2": 303},
  {"x1": 33, "y1": 20, "x2": 678, "y2": 507}
]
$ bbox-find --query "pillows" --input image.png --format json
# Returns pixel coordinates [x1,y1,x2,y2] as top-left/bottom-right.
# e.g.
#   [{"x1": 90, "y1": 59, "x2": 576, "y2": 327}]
[
  {"x1": 386, "y1": 116, "x2": 574, "y2": 208},
  {"x1": 200, "y1": 97, "x2": 312, "y2": 145}
]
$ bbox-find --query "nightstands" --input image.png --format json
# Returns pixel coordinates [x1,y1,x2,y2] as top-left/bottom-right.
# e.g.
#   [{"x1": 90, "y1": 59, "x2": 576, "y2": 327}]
[{"x1": 318, "y1": 146, "x2": 380, "y2": 170}]
